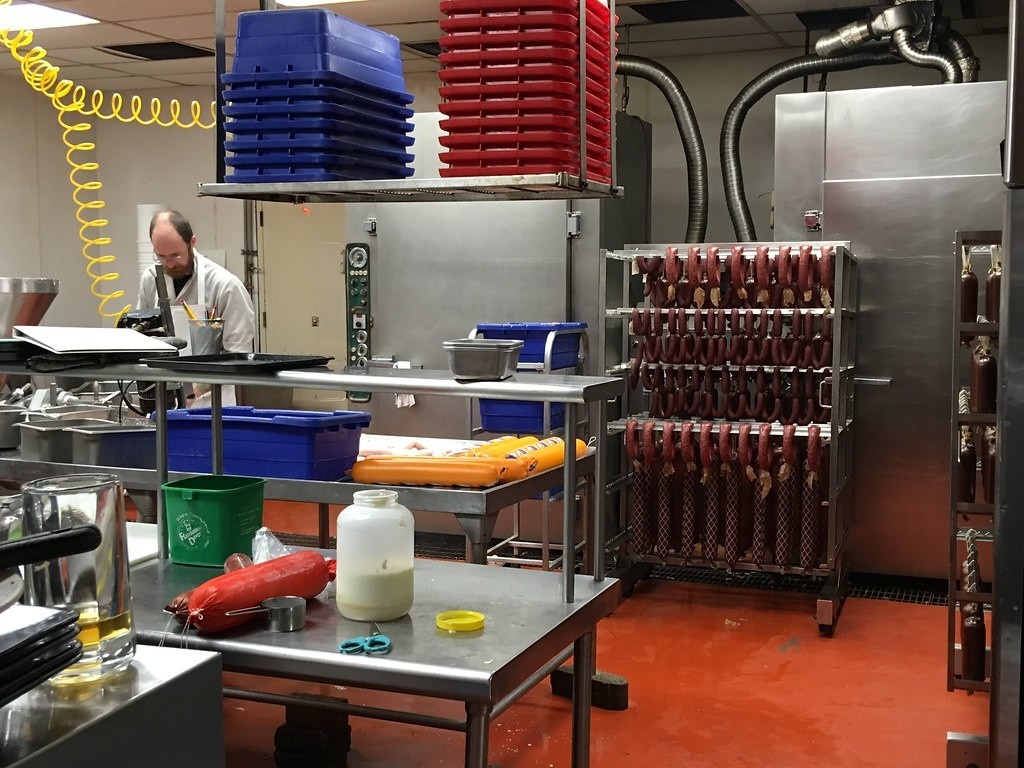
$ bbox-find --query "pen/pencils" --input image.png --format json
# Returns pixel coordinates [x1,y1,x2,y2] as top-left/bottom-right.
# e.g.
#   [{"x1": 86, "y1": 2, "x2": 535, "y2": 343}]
[
  {"x1": 209, "y1": 305, "x2": 217, "y2": 326},
  {"x1": 205, "y1": 310, "x2": 208, "y2": 326},
  {"x1": 180, "y1": 299, "x2": 201, "y2": 327}
]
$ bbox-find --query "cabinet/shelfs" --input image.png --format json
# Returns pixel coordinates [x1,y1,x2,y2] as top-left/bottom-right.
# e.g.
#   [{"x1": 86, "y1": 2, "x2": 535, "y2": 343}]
[
  {"x1": 2, "y1": 346, "x2": 625, "y2": 768},
  {"x1": 944, "y1": 228, "x2": 1006, "y2": 697},
  {"x1": 596, "y1": 240, "x2": 860, "y2": 642}
]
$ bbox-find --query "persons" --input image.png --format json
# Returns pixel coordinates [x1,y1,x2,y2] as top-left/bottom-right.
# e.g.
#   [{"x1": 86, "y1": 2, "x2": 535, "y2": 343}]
[{"x1": 136, "y1": 209, "x2": 257, "y2": 410}]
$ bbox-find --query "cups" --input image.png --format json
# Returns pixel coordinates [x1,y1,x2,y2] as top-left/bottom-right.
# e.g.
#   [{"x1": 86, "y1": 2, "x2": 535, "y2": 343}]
[{"x1": 19, "y1": 468, "x2": 138, "y2": 687}]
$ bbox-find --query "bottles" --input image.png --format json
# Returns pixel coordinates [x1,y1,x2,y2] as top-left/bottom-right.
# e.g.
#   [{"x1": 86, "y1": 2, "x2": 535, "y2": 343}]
[{"x1": 335, "y1": 490, "x2": 418, "y2": 624}]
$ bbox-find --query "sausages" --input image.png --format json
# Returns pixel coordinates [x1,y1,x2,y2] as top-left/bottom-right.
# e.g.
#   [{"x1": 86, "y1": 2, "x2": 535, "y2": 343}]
[{"x1": 165, "y1": 549, "x2": 336, "y2": 632}]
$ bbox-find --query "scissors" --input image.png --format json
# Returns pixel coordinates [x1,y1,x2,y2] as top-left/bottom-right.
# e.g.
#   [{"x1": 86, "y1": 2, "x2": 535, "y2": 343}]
[{"x1": 339, "y1": 621, "x2": 393, "y2": 657}]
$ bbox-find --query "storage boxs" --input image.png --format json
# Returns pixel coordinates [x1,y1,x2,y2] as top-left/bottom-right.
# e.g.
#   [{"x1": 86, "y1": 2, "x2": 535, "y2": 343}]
[
  {"x1": 442, "y1": 338, "x2": 524, "y2": 381},
  {"x1": 220, "y1": 7, "x2": 419, "y2": 185},
  {"x1": 435, "y1": 0, "x2": 621, "y2": 187},
  {"x1": 475, "y1": 322, "x2": 588, "y2": 434},
  {"x1": 159, "y1": 474, "x2": 266, "y2": 568},
  {"x1": 0, "y1": 394, "x2": 156, "y2": 470},
  {"x1": 151, "y1": 406, "x2": 371, "y2": 482}
]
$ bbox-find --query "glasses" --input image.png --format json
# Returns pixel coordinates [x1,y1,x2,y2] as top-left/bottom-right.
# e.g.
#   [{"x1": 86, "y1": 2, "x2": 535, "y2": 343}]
[{"x1": 152, "y1": 244, "x2": 190, "y2": 263}]
